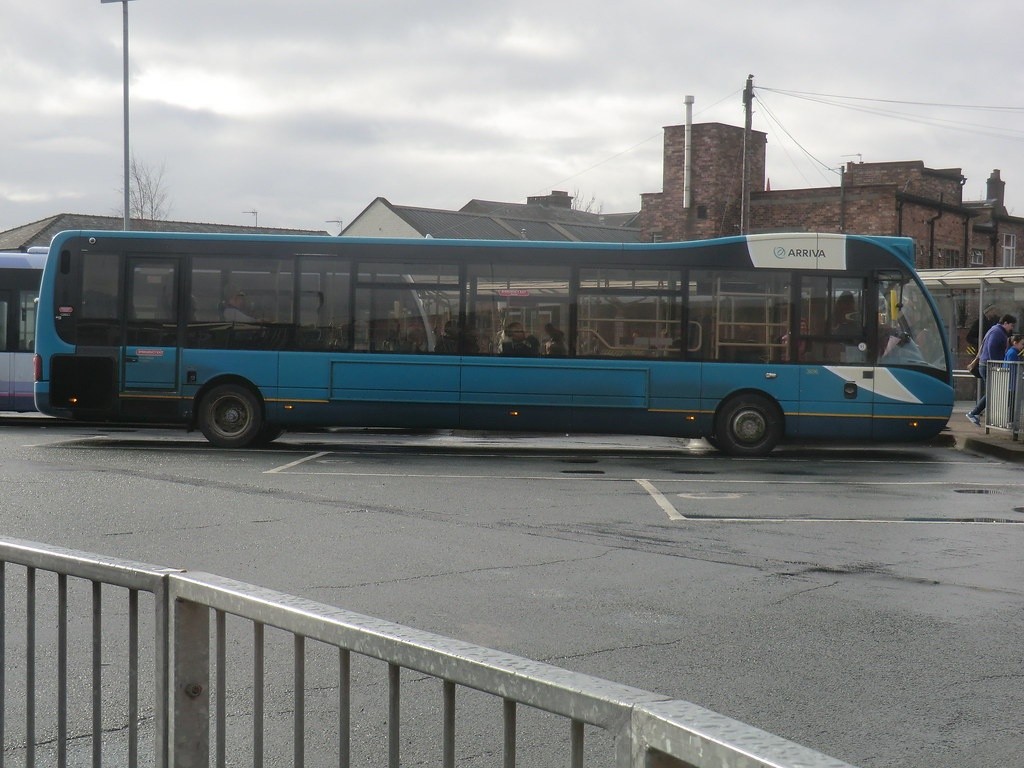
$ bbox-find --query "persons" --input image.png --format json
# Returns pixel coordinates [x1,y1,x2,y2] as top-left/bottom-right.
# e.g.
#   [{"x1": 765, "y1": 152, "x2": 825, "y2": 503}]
[
  {"x1": 966, "y1": 300, "x2": 1024, "y2": 428},
  {"x1": 174, "y1": 287, "x2": 869, "y2": 368}
]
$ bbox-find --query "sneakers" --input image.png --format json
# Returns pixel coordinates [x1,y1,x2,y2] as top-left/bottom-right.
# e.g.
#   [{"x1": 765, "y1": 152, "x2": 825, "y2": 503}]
[{"x1": 965, "y1": 410, "x2": 982, "y2": 428}]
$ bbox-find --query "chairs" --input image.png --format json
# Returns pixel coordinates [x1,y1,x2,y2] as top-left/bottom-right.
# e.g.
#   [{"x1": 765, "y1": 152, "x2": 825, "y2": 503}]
[
  {"x1": 218, "y1": 300, "x2": 228, "y2": 323},
  {"x1": 501, "y1": 340, "x2": 515, "y2": 353}
]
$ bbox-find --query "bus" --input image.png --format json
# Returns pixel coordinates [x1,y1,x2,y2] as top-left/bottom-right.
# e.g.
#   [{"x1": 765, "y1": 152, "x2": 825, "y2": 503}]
[
  {"x1": 36, "y1": 214, "x2": 955, "y2": 455},
  {"x1": 0, "y1": 242, "x2": 67, "y2": 414}
]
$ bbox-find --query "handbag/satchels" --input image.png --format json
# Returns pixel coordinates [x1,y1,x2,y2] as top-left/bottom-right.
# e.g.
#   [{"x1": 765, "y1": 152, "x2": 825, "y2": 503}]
[{"x1": 966, "y1": 338, "x2": 986, "y2": 379}]
